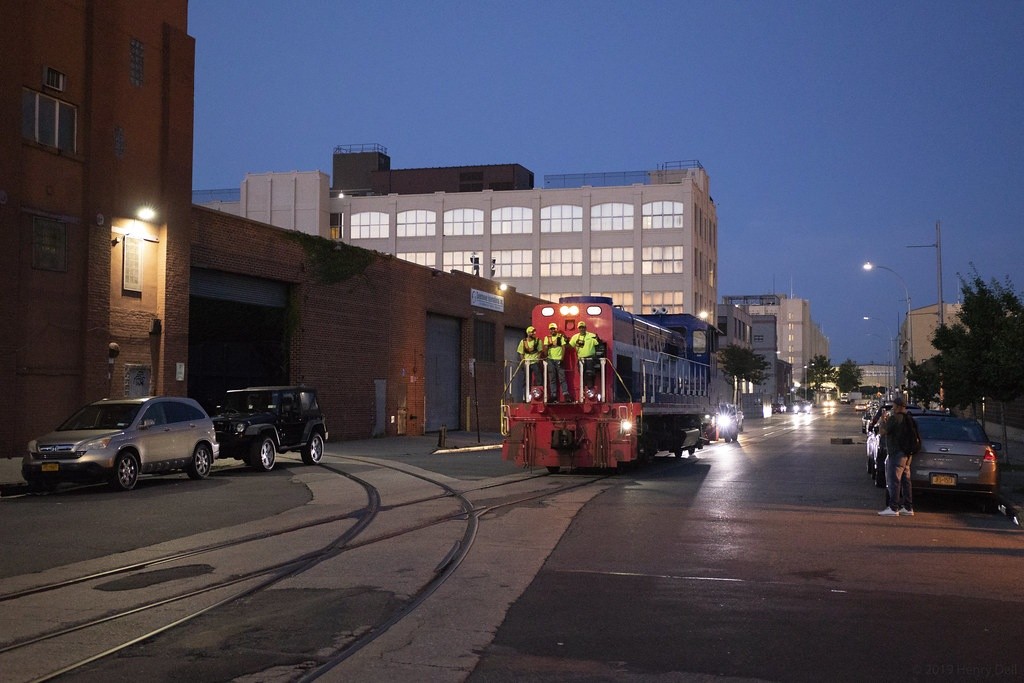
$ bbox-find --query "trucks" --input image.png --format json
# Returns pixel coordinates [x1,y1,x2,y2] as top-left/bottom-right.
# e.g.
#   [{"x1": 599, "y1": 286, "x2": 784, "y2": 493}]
[{"x1": 840, "y1": 392, "x2": 851, "y2": 405}]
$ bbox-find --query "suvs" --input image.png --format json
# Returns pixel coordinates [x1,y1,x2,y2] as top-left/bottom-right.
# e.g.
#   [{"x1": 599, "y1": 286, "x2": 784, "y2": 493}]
[
  {"x1": 210, "y1": 386, "x2": 329, "y2": 473},
  {"x1": 21, "y1": 395, "x2": 221, "y2": 493}
]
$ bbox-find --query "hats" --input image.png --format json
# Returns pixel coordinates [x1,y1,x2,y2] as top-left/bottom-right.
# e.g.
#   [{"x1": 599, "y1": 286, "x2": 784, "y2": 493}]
[
  {"x1": 548, "y1": 323, "x2": 558, "y2": 329},
  {"x1": 526, "y1": 326, "x2": 536, "y2": 334},
  {"x1": 889, "y1": 397, "x2": 907, "y2": 407},
  {"x1": 577, "y1": 321, "x2": 586, "y2": 328}
]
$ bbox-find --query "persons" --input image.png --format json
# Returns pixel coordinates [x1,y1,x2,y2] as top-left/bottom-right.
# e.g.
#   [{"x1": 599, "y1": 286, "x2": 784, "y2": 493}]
[
  {"x1": 541, "y1": 323, "x2": 570, "y2": 401},
  {"x1": 564, "y1": 322, "x2": 601, "y2": 400},
  {"x1": 517, "y1": 326, "x2": 544, "y2": 401},
  {"x1": 877, "y1": 397, "x2": 921, "y2": 516}
]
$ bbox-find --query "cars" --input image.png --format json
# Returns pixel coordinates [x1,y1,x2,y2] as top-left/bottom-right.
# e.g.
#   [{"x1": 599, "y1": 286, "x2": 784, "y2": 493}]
[
  {"x1": 771, "y1": 399, "x2": 813, "y2": 414},
  {"x1": 705, "y1": 404, "x2": 745, "y2": 444},
  {"x1": 884, "y1": 414, "x2": 1003, "y2": 510},
  {"x1": 854, "y1": 400, "x2": 948, "y2": 489}
]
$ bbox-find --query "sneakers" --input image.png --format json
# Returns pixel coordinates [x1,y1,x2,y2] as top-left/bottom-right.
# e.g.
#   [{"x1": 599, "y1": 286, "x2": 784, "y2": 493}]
[
  {"x1": 898, "y1": 508, "x2": 914, "y2": 516},
  {"x1": 877, "y1": 507, "x2": 899, "y2": 516}
]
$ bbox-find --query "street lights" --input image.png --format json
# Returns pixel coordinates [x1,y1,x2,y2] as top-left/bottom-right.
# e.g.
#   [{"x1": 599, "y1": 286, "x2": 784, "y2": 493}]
[
  {"x1": 863, "y1": 316, "x2": 896, "y2": 402},
  {"x1": 863, "y1": 260, "x2": 912, "y2": 405},
  {"x1": 803, "y1": 366, "x2": 807, "y2": 400}
]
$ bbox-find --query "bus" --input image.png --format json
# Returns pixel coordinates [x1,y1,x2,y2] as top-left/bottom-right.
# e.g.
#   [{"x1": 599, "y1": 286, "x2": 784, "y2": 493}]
[{"x1": 499, "y1": 296, "x2": 725, "y2": 477}]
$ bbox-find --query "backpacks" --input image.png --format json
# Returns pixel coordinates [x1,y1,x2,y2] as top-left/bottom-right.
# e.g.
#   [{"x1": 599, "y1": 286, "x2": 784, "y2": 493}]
[{"x1": 891, "y1": 411, "x2": 921, "y2": 455}]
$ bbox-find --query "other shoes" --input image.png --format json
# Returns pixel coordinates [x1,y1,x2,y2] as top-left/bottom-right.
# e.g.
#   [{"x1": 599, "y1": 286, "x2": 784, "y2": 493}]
[
  {"x1": 551, "y1": 397, "x2": 559, "y2": 404},
  {"x1": 564, "y1": 394, "x2": 573, "y2": 403}
]
